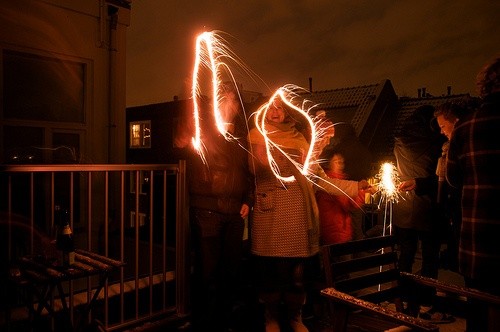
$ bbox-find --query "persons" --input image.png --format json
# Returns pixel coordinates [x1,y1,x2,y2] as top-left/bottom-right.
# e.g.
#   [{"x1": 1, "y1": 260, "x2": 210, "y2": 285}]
[
  {"x1": 171, "y1": 83, "x2": 250, "y2": 329},
  {"x1": 445, "y1": 58, "x2": 500, "y2": 332},
  {"x1": 250, "y1": 98, "x2": 460, "y2": 332}
]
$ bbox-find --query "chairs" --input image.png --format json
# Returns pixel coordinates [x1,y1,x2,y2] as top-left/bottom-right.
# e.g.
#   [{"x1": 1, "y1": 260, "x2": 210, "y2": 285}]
[{"x1": 321, "y1": 235, "x2": 500, "y2": 332}]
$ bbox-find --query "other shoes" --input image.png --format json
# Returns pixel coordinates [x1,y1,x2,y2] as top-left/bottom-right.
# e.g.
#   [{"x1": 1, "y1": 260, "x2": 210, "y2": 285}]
[{"x1": 419, "y1": 306, "x2": 455, "y2": 323}]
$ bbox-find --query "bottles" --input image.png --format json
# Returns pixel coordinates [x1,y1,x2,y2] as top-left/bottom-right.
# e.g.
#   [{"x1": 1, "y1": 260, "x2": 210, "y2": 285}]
[{"x1": 60, "y1": 211, "x2": 75, "y2": 265}]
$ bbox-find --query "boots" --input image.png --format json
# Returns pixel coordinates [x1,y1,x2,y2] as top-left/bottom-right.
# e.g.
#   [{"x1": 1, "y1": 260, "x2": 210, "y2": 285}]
[
  {"x1": 286, "y1": 292, "x2": 309, "y2": 332},
  {"x1": 264, "y1": 293, "x2": 281, "y2": 332}
]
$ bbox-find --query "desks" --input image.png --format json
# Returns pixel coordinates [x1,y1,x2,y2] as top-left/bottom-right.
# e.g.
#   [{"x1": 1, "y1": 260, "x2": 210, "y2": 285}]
[{"x1": 4, "y1": 249, "x2": 125, "y2": 332}]
